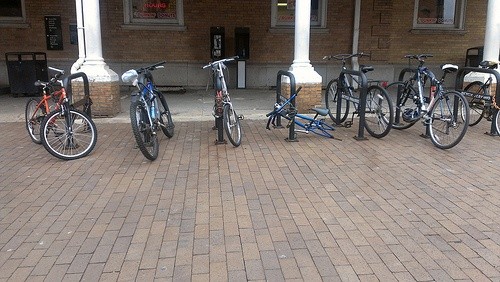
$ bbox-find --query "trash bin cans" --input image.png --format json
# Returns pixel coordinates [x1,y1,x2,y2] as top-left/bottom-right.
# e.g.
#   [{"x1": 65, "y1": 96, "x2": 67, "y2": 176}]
[
  {"x1": 464, "y1": 45, "x2": 484, "y2": 67},
  {"x1": 4, "y1": 50, "x2": 50, "y2": 98}
]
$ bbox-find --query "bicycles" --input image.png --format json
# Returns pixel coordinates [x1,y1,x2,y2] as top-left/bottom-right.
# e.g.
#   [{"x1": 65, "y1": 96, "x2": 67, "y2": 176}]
[
  {"x1": 24, "y1": 66, "x2": 98, "y2": 160},
  {"x1": 200, "y1": 55, "x2": 244, "y2": 147},
  {"x1": 265, "y1": 85, "x2": 335, "y2": 140},
  {"x1": 378, "y1": 53, "x2": 470, "y2": 149},
  {"x1": 459, "y1": 60, "x2": 500, "y2": 135},
  {"x1": 321, "y1": 52, "x2": 394, "y2": 139},
  {"x1": 121, "y1": 61, "x2": 175, "y2": 161}
]
